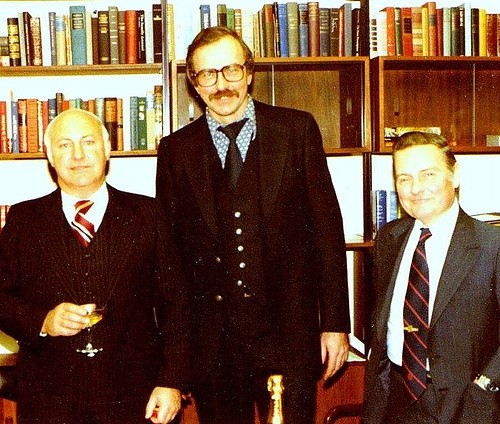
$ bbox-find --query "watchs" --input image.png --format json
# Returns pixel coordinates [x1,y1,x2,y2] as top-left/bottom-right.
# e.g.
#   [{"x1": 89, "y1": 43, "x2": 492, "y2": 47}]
[{"x1": 475, "y1": 373, "x2": 500, "y2": 392}]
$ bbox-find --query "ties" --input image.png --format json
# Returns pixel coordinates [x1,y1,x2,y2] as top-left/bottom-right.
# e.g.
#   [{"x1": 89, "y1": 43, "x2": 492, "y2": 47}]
[
  {"x1": 217, "y1": 118, "x2": 248, "y2": 186},
  {"x1": 400, "y1": 228, "x2": 432, "y2": 404},
  {"x1": 69, "y1": 200, "x2": 95, "y2": 248}
]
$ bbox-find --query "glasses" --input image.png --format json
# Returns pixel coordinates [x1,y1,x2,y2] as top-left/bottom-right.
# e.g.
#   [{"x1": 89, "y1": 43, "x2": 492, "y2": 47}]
[{"x1": 190, "y1": 61, "x2": 247, "y2": 87}]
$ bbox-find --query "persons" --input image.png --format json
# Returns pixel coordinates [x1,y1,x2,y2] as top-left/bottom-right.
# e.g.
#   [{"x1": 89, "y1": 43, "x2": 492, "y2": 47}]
[
  {"x1": 359, "y1": 131, "x2": 500, "y2": 424},
  {"x1": 0, "y1": 108, "x2": 195, "y2": 424},
  {"x1": 155, "y1": 26, "x2": 352, "y2": 424}
]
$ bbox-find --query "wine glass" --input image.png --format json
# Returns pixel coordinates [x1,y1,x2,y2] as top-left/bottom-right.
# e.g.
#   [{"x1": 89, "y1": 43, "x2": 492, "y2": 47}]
[{"x1": 77, "y1": 306, "x2": 106, "y2": 357}]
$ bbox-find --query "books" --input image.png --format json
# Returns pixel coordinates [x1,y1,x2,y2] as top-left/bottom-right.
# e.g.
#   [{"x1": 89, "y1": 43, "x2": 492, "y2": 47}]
[
  {"x1": 369, "y1": 188, "x2": 500, "y2": 233},
  {"x1": 1, "y1": 4, "x2": 165, "y2": 65},
  {"x1": 198, "y1": 2, "x2": 361, "y2": 58},
  {"x1": 368, "y1": 2, "x2": 500, "y2": 60},
  {"x1": 0, "y1": 85, "x2": 164, "y2": 153},
  {"x1": 1, "y1": 205, "x2": 12, "y2": 230},
  {"x1": 383, "y1": 127, "x2": 443, "y2": 143}
]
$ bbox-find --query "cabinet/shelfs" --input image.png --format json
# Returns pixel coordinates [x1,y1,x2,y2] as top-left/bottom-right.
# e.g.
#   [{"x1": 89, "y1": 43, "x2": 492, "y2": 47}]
[
  {"x1": 371, "y1": 0, "x2": 500, "y2": 243},
  {"x1": 2, "y1": 0, "x2": 499, "y2": 338}
]
